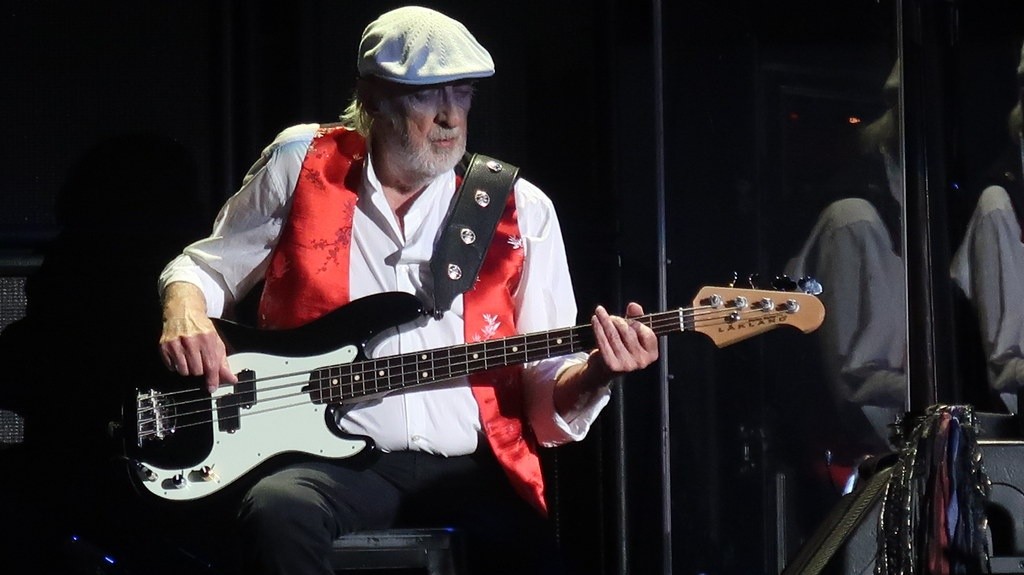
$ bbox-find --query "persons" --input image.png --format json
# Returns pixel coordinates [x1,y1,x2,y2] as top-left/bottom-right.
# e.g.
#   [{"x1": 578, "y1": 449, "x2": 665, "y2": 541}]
[
  {"x1": 778, "y1": 88, "x2": 905, "y2": 538},
  {"x1": 950, "y1": 94, "x2": 1024, "y2": 417},
  {"x1": 159, "y1": 5, "x2": 658, "y2": 575}
]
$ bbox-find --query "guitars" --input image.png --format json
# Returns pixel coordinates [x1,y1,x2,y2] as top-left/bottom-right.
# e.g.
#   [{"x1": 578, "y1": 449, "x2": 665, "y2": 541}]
[{"x1": 113, "y1": 268, "x2": 829, "y2": 513}]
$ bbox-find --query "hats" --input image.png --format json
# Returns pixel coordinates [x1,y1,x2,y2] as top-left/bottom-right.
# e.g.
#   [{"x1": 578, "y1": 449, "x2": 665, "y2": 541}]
[
  {"x1": 357, "y1": 6, "x2": 495, "y2": 84},
  {"x1": 884, "y1": 58, "x2": 898, "y2": 88},
  {"x1": 1018, "y1": 44, "x2": 1024, "y2": 74}
]
